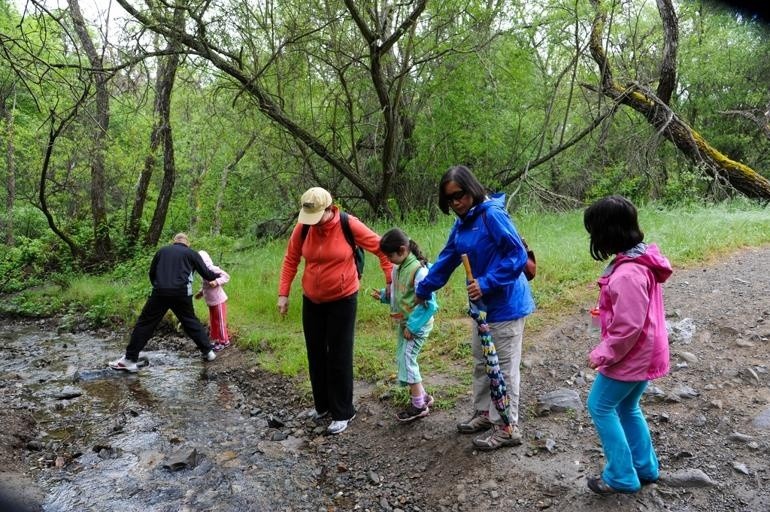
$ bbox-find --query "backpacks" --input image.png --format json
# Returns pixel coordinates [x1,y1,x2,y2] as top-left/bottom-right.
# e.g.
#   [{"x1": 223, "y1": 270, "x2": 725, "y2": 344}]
[
  {"x1": 482, "y1": 205, "x2": 537, "y2": 281},
  {"x1": 410, "y1": 257, "x2": 437, "y2": 299},
  {"x1": 296, "y1": 211, "x2": 365, "y2": 283}
]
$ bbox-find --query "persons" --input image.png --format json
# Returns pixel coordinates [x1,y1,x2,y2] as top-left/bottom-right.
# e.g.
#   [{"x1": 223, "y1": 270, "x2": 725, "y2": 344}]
[
  {"x1": 108, "y1": 231, "x2": 223, "y2": 374},
  {"x1": 577, "y1": 193, "x2": 672, "y2": 497},
  {"x1": 193, "y1": 248, "x2": 234, "y2": 350},
  {"x1": 372, "y1": 225, "x2": 438, "y2": 424},
  {"x1": 274, "y1": 187, "x2": 392, "y2": 435},
  {"x1": 415, "y1": 164, "x2": 537, "y2": 451}
]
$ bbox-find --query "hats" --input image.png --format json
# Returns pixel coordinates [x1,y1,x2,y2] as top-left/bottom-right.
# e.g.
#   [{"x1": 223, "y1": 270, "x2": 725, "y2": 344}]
[
  {"x1": 173, "y1": 232, "x2": 190, "y2": 247},
  {"x1": 298, "y1": 187, "x2": 332, "y2": 226}
]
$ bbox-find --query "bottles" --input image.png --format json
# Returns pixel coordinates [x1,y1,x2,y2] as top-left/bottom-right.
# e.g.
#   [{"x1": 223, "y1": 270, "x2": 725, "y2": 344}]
[
  {"x1": 386, "y1": 311, "x2": 402, "y2": 338},
  {"x1": 587, "y1": 308, "x2": 601, "y2": 338}
]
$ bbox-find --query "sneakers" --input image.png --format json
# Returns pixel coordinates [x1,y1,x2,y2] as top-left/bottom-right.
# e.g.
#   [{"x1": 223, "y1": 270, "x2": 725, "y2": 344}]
[
  {"x1": 209, "y1": 339, "x2": 230, "y2": 351},
  {"x1": 457, "y1": 414, "x2": 492, "y2": 434},
  {"x1": 587, "y1": 473, "x2": 640, "y2": 496},
  {"x1": 471, "y1": 425, "x2": 522, "y2": 451},
  {"x1": 395, "y1": 403, "x2": 429, "y2": 421},
  {"x1": 327, "y1": 412, "x2": 357, "y2": 434},
  {"x1": 204, "y1": 350, "x2": 214, "y2": 361},
  {"x1": 423, "y1": 393, "x2": 434, "y2": 409},
  {"x1": 109, "y1": 356, "x2": 139, "y2": 373},
  {"x1": 313, "y1": 406, "x2": 330, "y2": 419}
]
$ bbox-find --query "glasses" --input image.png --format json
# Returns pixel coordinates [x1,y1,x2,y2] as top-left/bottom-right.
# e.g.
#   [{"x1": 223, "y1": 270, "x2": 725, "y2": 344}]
[{"x1": 446, "y1": 190, "x2": 468, "y2": 202}]
